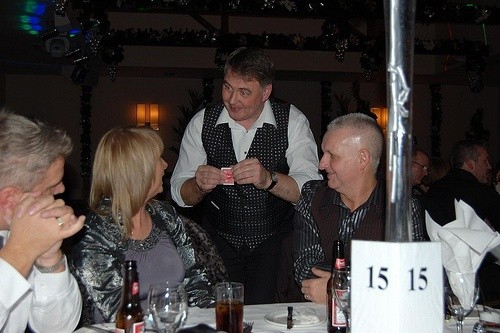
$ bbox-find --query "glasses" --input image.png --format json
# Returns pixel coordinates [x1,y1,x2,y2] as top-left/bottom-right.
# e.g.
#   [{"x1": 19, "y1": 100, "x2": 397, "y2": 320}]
[{"x1": 412, "y1": 161, "x2": 429, "y2": 171}]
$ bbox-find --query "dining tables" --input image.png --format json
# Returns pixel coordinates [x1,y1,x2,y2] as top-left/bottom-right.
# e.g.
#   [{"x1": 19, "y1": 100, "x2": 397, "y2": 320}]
[{"x1": 74, "y1": 303, "x2": 493, "y2": 333}]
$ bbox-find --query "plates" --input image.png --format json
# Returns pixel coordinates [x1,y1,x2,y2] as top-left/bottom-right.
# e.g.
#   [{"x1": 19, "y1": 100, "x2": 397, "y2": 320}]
[
  {"x1": 72, "y1": 322, "x2": 116, "y2": 333},
  {"x1": 264, "y1": 306, "x2": 328, "y2": 328}
]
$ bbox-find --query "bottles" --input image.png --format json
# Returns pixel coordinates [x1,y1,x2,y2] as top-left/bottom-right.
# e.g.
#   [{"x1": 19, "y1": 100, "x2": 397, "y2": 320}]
[
  {"x1": 116, "y1": 260, "x2": 145, "y2": 333},
  {"x1": 326, "y1": 239, "x2": 349, "y2": 333}
]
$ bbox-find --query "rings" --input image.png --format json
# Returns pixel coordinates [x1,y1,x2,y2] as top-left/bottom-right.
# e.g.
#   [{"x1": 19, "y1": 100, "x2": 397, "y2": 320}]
[
  {"x1": 55, "y1": 216, "x2": 64, "y2": 229},
  {"x1": 306, "y1": 294, "x2": 309, "y2": 301}
]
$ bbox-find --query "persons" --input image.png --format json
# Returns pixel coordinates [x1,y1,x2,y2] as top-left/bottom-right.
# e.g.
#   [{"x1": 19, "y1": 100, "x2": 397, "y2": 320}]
[
  {"x1": 422, "y1": 141, "x2": 500, "y2": 304},
  {"x1": 169, "y1": 48, "x2": 324, "y2": 304},
  {"x1": 68, "y1": 126, "x2": 230, "y2": 324},
  {"x1": 292, "y1": 113, "x2": 422, "y2": 302},
  {"x1": 412, "y1": 147, "x2": 433, "y2": 195},
  {"x1": 0, "y1": 108, "x2": 86, "y2": 333}
]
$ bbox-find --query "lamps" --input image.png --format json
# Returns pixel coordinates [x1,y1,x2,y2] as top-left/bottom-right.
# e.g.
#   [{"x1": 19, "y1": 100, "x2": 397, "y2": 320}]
[
  {"x1": 370, "y1": 107, "x2": 388, "y2": 132},
  {"x1": 136, "y1": 102, "x2": 159, "y2": 133}
]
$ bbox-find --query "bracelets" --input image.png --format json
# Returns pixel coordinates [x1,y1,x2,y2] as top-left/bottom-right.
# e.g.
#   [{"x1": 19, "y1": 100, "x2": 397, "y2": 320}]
[{"x1": 34, "y1": 253, "x2": 68, "y2": 273}]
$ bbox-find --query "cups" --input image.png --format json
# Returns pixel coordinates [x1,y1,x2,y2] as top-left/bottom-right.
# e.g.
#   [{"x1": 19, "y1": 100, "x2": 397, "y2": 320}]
[
  {"x1": 215, "y1": 282, "x2": 244, "y2": 333},
  {"x1": 147, "y1": 281, "x2": 188, "y2": 333}
]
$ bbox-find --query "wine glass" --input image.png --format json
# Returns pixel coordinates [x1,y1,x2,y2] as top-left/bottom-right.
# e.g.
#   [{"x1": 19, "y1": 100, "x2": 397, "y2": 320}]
[
  {"x1": 330, "y1": 266, "x2": 351, "y2": 333},
  {"x1": 443, "y1": 271, "x2": 480, "y2": 333}
]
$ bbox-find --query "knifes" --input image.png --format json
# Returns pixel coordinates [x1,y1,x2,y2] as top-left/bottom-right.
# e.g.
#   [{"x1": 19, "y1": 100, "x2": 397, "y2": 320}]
[{"x1": 287, "y1": 306, "x2": 293, "y2": 329}]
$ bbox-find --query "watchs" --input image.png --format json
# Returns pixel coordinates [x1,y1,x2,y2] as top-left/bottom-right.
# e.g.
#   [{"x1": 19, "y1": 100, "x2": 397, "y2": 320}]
[{"x1": 264, "y1": 171, "x2": 279, "y2": 191}]
